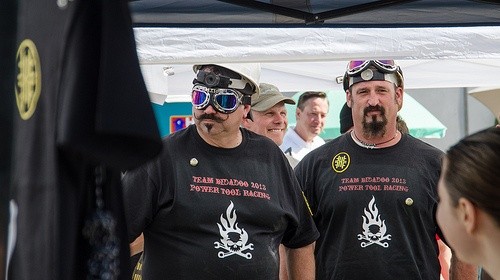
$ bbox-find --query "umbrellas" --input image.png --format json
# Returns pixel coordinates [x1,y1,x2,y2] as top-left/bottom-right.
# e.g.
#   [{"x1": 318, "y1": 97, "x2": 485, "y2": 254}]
[{"x1": 260, "y1": 87, "x2": 446, "y2": 141}]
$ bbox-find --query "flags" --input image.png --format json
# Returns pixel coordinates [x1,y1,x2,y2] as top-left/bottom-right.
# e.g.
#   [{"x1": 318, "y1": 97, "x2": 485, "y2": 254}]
[{"x1": 0, "y1": 0, "x2": 163, "y2": 280}]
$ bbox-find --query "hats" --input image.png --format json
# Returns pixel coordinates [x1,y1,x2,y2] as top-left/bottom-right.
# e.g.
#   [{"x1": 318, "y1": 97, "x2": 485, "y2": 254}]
[
  {"x1": 193, "y1": 64, "x2": 260, "y2": 100},
  {"x1": 343, "y1": 63, "x2": 405, "y2": 92},
  {"x1": 251, "y1": 82, "x2": 296, "y2": 112}
]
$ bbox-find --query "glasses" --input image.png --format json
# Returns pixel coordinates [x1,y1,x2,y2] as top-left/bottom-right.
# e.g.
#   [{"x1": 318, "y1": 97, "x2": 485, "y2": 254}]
[
  {"x1": 191, "y1": 84, "x2": 252, "y2": 114},
  {"x1": 347, "y1": 60, "x2": 398, "y2": 76}
]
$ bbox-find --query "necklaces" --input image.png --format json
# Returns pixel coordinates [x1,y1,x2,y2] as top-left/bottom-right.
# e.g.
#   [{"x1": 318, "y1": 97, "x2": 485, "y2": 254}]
[
  {"x1": 206, "y1": 134, "x2": 238, "y2": 147},
  {"x1": 354, "y1": 130, "x2": 398, "y2": 146}
]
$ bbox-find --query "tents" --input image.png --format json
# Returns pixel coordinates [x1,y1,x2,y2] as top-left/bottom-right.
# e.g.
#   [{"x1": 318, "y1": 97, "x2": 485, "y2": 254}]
[{"x1": 0, "y1": 0, "x2": 500, "y2": 103}]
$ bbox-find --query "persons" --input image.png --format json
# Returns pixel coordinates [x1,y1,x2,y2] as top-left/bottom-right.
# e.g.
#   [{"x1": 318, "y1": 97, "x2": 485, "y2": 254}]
[
  {"x1": 396, "y1": 116, "x2": 452, "y2": 280},
  {"x1": 240, "y1": 84, "x2": 297, "y2": 147},
  {"x1": 120, "y1": 63, "x2": 320, "y2": 280},
  {"x1": 436, "y1": 127, "x2": 500, "y2": 280},
  {"x1": 280, "y1": 91, "x2": 329, "y2": 161},
  {"x1": 340, "y1": 103, "x2": 354, "y2": 136},
  {"x1": 278, "y1": 60, "x2": 479, "y2": 280}
]
